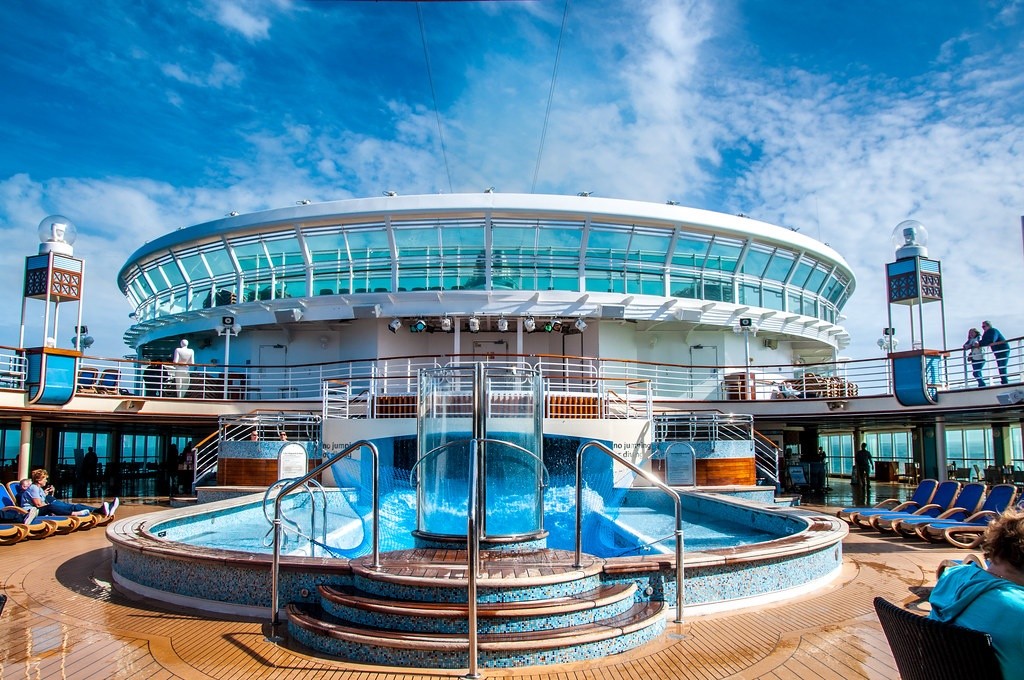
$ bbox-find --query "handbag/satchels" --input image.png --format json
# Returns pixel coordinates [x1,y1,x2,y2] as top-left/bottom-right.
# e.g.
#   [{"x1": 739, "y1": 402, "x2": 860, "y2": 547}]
[{"x1": 968, "y1": 352, "x2": 973, "y2": 361}]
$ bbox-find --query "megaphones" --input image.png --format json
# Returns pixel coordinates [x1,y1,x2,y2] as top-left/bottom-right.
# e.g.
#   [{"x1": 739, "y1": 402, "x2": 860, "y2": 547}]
[
  {"x1": 75, "y1": 325, "x2": 88, "y2": 334},
  {"x1": 884, "y1": 328, "x2": 895, "y2": 335},
  {"x1": 740, "y1": 318, "x2": 752, "y2": 328},
  {"x1": 222, "y1": 316, "x2": 235, "y2": 327}
]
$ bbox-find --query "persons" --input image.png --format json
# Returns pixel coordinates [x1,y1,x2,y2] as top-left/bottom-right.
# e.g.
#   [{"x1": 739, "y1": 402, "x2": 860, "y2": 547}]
[
  {"x1": 140, "y1": 360, "x2": 170, "y2": 396},
  {"x1": 976, "y1": 321, "x2": 1010, "y2": 385},
  {"x1": 170, "y1": 444, "x2": 179, "y2": 476},
  {"x1": 963, "y1": 328, "x2": 985, "y2": 387},
  {"x1": 856, "y1": 443, "x2": 874, "y2": 490},
  {"x1": 173, "y1": 340, "x2": 194, "y2": 399},
  {"x1": 819, "y1": 447, "x2": 828, "y2": 487},
  {"x1": 251, "y1": 431, "x2": 258, "y2": 441},
  {"x1": 780, "y1": 381, "x2": 821, "y2": 398},
  {"x1": 182, "y1": 441, "x2": 192, "y2": 459},
  {"x1": 926, "y1": 506, "x2": 1024, "y2": 680},
  {"x1": 280, "y1": 432, "x2": 286, "y2": 441},
  {"x1": 17, "y1": 470, "x2": 119, "y2": 518},
  {"x1": 84, "y1": 447, "x2": 98, "y2": 486}
]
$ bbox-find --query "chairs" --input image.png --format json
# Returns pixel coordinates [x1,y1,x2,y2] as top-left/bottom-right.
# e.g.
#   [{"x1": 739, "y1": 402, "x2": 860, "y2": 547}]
[
  {"x1": 836, "y1": 479, "x2": 1024, "y2": 549},
  {"x1": 0, "y1": 481, "x2": 116, "y2": 545},
  {"x1": 77, "y1": 367, "x2": 121, "y2": 396},
  {"x1": 782, "y1": 372, "x2": 858, "y2": 397},
  {"x1": 721, "y1": 376, "x2": 742, "y2": 400},
  {"x1": 874, "y1": 553, "x2": 1004, "y2": 680}
]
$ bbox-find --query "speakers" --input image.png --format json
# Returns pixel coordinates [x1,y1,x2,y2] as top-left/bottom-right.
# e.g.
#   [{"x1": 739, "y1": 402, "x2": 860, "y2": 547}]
[
  {"x1": 597, "y1": 305, "x2": 624, "y2": 318},
  {"x1": 353, "y1": 304, "x2": 381, "y2": 318},
  {"x1": 275, "y1": 308, "x2": 302, "y2": 323},
  {"x1": 996, "y1": 390, "x2": 1021, "y2": 404},
  {"x1": 675, "y1": 309, "x2": 702, "y2": 323}
]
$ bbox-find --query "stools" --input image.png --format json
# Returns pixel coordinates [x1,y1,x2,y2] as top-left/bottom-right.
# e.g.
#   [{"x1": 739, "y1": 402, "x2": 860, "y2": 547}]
[
  {"x1": 242, "y1": 387, "x2": 262, "y2": 400},
  {"x1": 281, "y1": 386, "x2": 298, "y2": 398}
]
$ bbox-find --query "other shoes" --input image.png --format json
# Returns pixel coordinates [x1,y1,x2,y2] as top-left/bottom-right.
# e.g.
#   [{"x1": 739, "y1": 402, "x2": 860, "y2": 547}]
[
  {"x1": 867, "y1": 485, "x2": 871, "y2": 488},
  {"x1": 108, "y1": 497, "x2": 119, "y2": 517},
  {"x1": 862, "y1": 485, "x2": 865, "y2": 488},
  {"x1": 100, "y1": 502, "x2": 110, "y2": 517}
]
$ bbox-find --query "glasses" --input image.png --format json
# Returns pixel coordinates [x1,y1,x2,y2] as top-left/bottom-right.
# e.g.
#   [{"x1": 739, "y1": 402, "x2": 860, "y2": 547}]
[{"x1": 981, "y1": 326, "x2": 986, "y2": 328}]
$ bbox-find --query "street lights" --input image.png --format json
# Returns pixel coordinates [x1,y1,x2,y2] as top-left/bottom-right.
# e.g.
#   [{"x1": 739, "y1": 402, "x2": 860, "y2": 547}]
[
  {"x1": 215, "y1": 317, "x2": 241, "y2": 400},
  {"x1": 733, "y1": 317, "x2": 758, "y2": 399},
  {"x1": 878, "y1": 328, "x2": 898, "y2": 394},
  {"x1": 71, "y1": 325, "x2": 94, "y2": 369}
]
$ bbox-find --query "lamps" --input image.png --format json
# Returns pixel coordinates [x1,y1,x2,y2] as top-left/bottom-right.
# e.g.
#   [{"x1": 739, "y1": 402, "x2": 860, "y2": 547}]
[
  {"x1": 414, "y1": 318, "x2": 427, "y2": 332},
  {"x1": 499, "y1": 319, "x2": 508, "y2": 331},
  {"x1": 388, "y1": 317, "x2": 402, "y2": 334},
  {"x1": 551, "y1": 317, "x2": 563, "y2": 333},
  {"x1": 524, "y1": 319, "x2": 535, "y2": 333},
  {"x1": 469, "y1": 318, "x2": 479, "y2": 331},
  {"x1": 546, "y1": 323, "x2": 553, "y2": 333},
  {"x1": 575, "y1": 320, "x2": 588, "y2": 332},
  {"x1": 441, "y1": 317, "x2": 451, "y2": 331}
]
